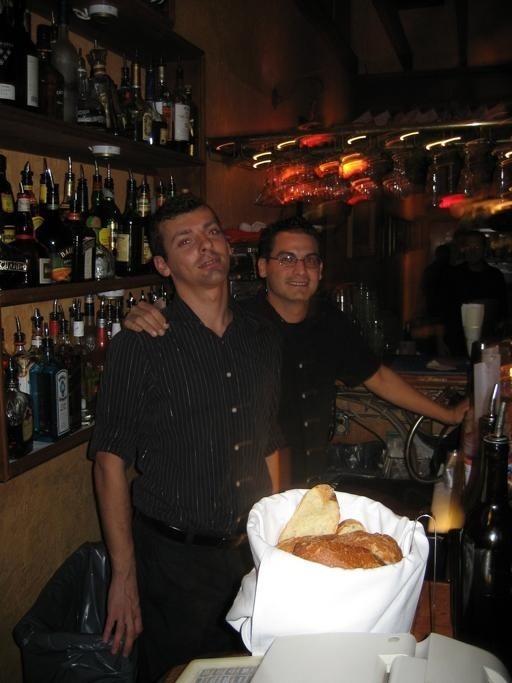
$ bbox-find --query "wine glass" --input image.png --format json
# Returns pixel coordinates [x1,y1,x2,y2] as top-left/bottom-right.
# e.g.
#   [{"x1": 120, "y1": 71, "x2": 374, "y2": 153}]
[
  {"x1": 229, "y1": 249, "x2": 266, "y2": 298},
  {"x1": 258, "y1": 144, "x2": 512, "y2": 209}
]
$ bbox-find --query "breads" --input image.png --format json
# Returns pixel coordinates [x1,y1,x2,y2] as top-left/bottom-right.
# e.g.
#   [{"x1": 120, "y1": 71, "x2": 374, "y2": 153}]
[{"x1": 275, "y1": 484, "x2": 403, "y2": 568}]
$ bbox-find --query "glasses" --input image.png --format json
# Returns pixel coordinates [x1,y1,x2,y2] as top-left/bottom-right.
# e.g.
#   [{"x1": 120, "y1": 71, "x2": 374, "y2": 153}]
[{"x1": 269, "y1": 254, "x2": 322, "y2": 269}]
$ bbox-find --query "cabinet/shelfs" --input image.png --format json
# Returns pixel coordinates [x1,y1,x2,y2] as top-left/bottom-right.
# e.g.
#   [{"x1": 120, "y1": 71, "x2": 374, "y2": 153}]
[{"x1": 0, "y1": 0, "x2": 207, "y2": 484}]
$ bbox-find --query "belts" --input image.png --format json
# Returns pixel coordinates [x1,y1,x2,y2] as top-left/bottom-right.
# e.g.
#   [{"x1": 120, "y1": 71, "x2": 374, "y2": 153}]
[{"x1": 134, "y1": 514, "x2": 249, "y2": 549}]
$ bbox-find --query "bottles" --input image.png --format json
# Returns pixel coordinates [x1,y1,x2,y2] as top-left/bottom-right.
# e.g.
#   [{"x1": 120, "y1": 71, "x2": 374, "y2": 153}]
[
  {"x1": 451, "y1": 402, "x2": 512, "y2": 665},
  {"x1": 0, "y1": 151, "x2": 180, "y2": 289},
  {"x1": 0, "y1": 287, "x2": 173, "y2": 459},
  {"x1": 0, "y1": 1, "x2": 199, "y2": 154},
  {"x1": 336, "y1": 283, "x2": 399, "y2": 367}
]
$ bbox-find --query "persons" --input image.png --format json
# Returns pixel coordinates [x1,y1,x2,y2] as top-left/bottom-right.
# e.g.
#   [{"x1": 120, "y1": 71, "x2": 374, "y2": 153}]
[
  {"x1": 413, "y1": 227, "x2": 508, "y2": 353},
  {"x1": 87, "y1": 192, "x2": 283, "y2": 683},
  {"x1": 122, "y1": 220, "x2": 469, "y2": 482}
]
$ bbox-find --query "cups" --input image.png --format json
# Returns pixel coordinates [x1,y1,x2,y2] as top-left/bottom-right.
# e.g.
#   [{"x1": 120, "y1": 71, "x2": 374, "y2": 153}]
[{"x1": 461, "y1": 304, "x2": 484, "y2": 358}]
